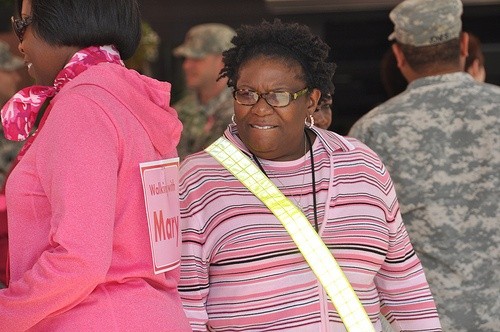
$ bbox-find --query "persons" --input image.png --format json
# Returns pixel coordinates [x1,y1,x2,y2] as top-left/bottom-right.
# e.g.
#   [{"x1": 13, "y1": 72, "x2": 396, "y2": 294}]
[
  {"x1": 464, "y1": 34, "x2": 486, "y2": 82},
  {"x1": 0, "y1": 0, "x2": 192, "y2": 332},
  {"x1": 0, "y1": 41, "x2": 36, "y2": 186},
  {"x1": 171, "y1": 23, "x2": 238, "y2": 163},
  {"x1": 347, "y1": 0, "x2": 500, "y2": 332},
  {"x1": 177, "y1": 18, "x2": 443, "y2": 332},
  {"x1": 313, "y1": 63, "x2": 337, "y2": 130}
]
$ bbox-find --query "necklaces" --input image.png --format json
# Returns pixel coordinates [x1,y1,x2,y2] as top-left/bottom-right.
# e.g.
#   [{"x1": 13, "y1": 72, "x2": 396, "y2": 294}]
[
  {"x1": 235, "y1": 129, "x2": 319, "y2": 233},
  {"x1": 257, "y1": 132, "x2": 306, "y2": 213}
]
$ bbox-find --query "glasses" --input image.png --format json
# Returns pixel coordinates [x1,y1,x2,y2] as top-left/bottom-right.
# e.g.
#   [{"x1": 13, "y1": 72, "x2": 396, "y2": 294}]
[
  {"x1": 232, "y1": 86, "x2": 310, "y2": 107},
  {"x1": 11, "y1": 14, "x2": 34, "y2": 42}
]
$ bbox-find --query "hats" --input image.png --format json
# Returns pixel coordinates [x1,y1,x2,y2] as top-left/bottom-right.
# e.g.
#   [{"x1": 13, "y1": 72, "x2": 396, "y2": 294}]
[
  {"x1": 388, "y1": 0, "x2": 463, "y2": 48},
  {"x1": 173, "y1": 24, "x2": 238, "y2": 58}
]
